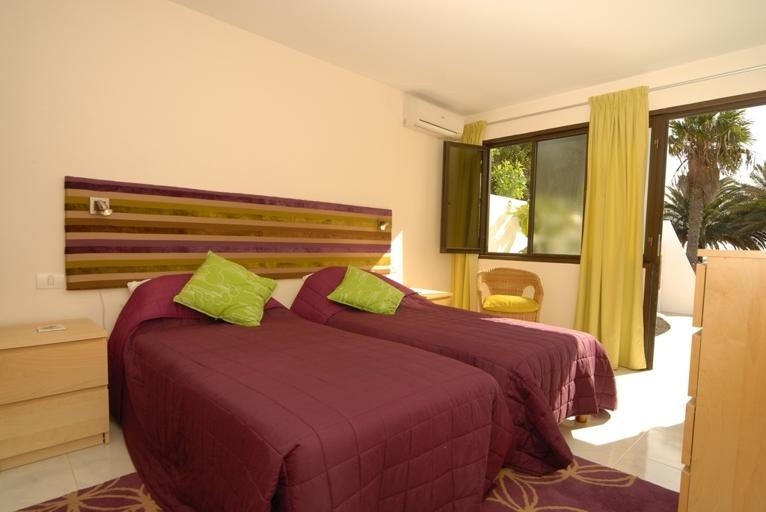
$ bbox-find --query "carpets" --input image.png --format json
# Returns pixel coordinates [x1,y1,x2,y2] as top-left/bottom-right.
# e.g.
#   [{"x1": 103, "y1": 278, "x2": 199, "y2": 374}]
[
  {"x1": 13, "y1": 470, "x2": 167, "y2": 512},
  {"x1": 482, "y1": 452, "x2": 682, "y2": 512}
]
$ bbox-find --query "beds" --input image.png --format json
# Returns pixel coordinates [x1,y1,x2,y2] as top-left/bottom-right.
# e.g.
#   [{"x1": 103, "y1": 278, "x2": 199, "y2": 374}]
[
  {"x1": 288, "y1": 266, "x2": 619, "y2": 478},
  {"x1": 107, "y1": 278, "x2": 518, "y2": 510}
]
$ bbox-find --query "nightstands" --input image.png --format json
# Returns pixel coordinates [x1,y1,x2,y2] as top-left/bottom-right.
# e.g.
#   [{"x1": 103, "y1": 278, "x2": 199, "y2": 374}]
[{"x1": 0, "y1": 317, "x2": 111, "y2": 472}]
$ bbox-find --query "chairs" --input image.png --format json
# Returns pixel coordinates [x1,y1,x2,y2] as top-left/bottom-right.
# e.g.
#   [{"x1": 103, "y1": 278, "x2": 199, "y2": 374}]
[{"x1": 474, "y1": 267, "x2": 544, "y2": 324}]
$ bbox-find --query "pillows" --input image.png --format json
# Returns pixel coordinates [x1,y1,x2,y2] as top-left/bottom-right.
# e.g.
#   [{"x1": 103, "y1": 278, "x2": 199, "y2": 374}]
[
  {"x1": 173, "y1": 248, "x2": 279, "y2": 327},
  {"x1": 327, "y1": 263, "x2": 405, "y2": 316}
]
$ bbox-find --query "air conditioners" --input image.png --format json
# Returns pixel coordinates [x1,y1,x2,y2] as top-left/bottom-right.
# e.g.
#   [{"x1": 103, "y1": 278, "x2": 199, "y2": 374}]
[{"x1": 403, "y1": 92, "x2": 465, "y2": 142}]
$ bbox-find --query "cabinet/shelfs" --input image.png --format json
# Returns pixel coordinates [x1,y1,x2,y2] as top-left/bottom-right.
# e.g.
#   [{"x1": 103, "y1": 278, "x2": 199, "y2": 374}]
[{"x1": 677, "y1": 249, "x2": 766, "y2": 510}]
